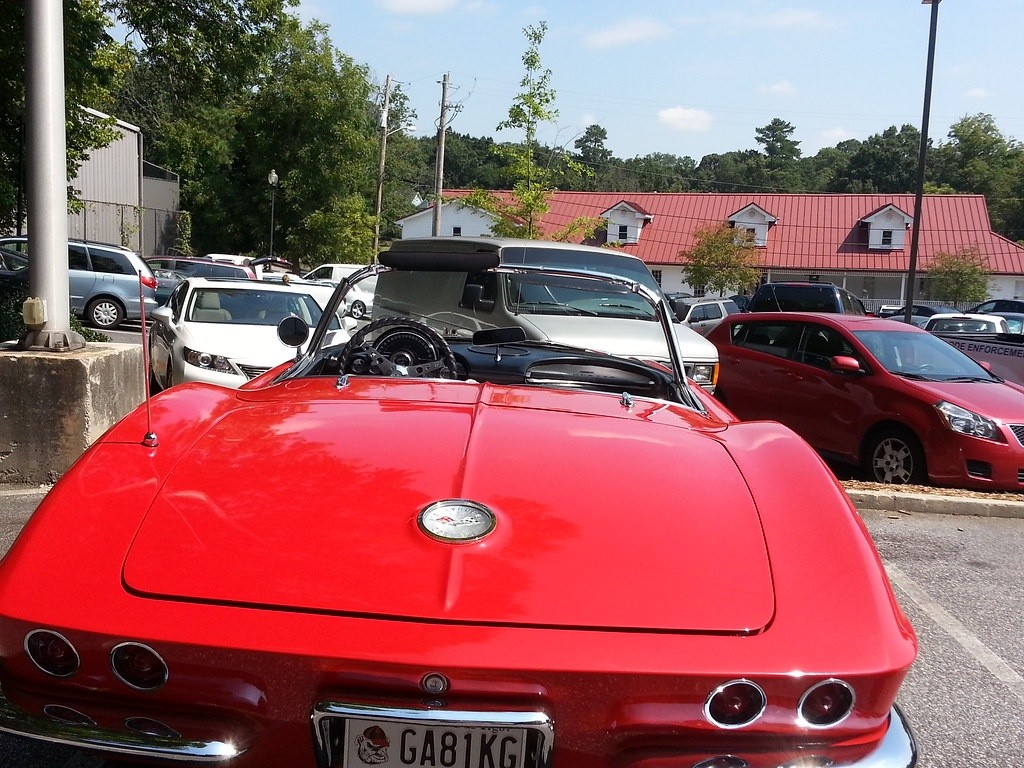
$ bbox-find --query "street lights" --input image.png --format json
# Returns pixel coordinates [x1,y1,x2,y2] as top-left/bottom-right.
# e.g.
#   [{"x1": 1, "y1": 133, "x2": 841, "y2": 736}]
[{"x1": 268, "y1": 168, "x2": 278, "y2": 258}]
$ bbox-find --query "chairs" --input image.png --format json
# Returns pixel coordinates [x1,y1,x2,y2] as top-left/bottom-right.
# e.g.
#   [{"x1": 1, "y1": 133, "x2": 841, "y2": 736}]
[
  {"x1": 257, "y1": 294, "x2": 293, "y2": 321},
  {"x1": 193, "y1": 291, "x2": 233, "y2": 323},
  {"x1": 807, "y1": 335, "x2": 843, "y2": 375}
]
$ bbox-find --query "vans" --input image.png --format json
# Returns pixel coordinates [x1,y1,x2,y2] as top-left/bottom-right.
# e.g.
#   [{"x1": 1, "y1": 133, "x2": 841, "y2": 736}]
[
  {"x1": 302, "y1": 263, "x2": 383, "y2": 319},
  {"x1": 368, "y1": 236, "x2": 720, "y2": 397}
]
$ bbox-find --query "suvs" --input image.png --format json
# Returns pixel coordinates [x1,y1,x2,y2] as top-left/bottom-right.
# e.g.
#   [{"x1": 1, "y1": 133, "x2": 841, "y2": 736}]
[
  {"x1": 0, "y1": 238, "x2": 159, "y2": 330},
  {"x1": 142, "y1": 254, "x2": 258, "y2": 308}
]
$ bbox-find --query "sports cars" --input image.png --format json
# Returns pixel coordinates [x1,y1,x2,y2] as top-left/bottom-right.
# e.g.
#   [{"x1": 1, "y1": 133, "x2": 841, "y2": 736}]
[{"x1": 0, "y1": 255, "x2": 921, "y2": 768}]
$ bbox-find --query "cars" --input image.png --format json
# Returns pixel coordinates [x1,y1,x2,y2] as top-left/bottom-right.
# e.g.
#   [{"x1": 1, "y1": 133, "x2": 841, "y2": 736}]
[
  {"x1": 145, "y1": 277, "x2": 358, "y2": 391},
  {"x1": 202, "y1": 253, "x2": 347, "y2": 318},
  {"x1": 0, "y1": 246, "x2": 29, "y2": 295},
  {"x1": 702, "y1": 311, "x2": 1024, "y2": 494},
  {"x1": 661, "y1": 280, "x2": 1024, "y2": 390}
]
